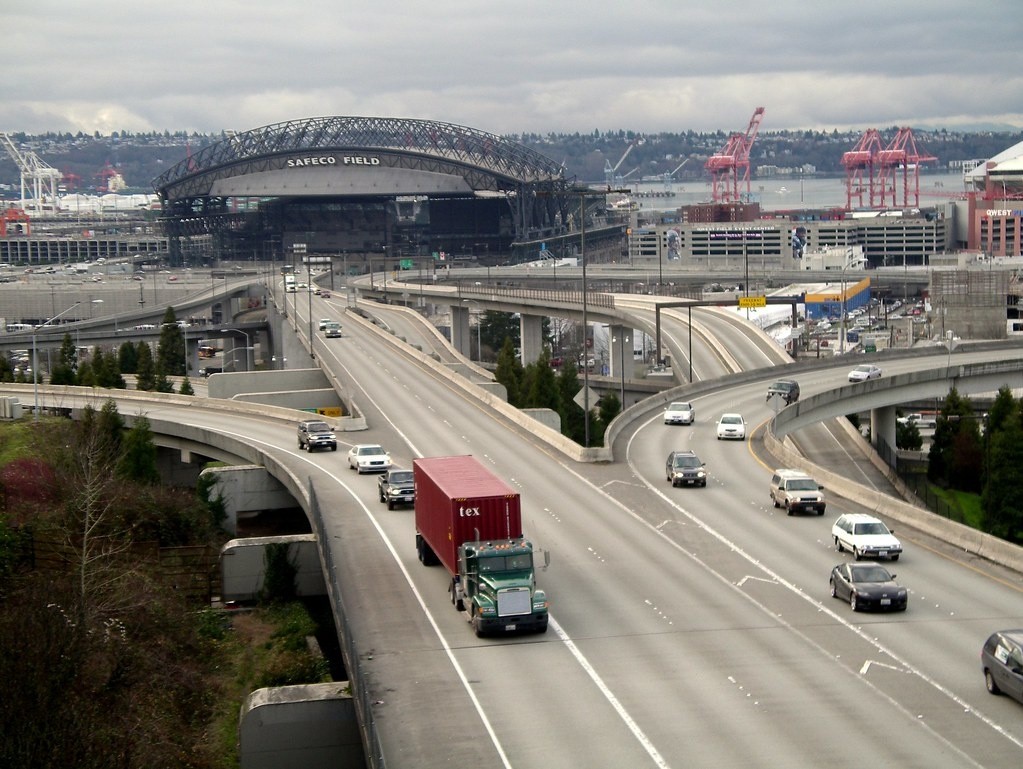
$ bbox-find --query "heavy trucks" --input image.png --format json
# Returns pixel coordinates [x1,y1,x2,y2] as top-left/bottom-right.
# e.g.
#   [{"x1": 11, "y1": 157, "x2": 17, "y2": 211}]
[{"x1": 411, "y1": 455, "x2": 550, "y2": 640}]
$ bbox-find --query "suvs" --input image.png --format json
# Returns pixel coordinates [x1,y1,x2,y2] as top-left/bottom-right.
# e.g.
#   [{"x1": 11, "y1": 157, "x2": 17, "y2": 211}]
[
  {"x1": 768, "y1": 467, "x2": 827, "y2": 515},
  {"x1": 297, "y1": 419, "x2": 337, "y2": 453},
  {"x1": 664, "y1": 449, "x2": 707, "y2": 491}
]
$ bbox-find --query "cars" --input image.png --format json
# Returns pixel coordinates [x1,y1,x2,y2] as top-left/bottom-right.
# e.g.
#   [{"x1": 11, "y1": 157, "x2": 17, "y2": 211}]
[
  {"x1": 715, "y1": 414, "x2": 749, "y2": 442},
  {"x1": 280, "y1": 264, "x2": 333, "y2": 300},
  {"x1": 320, "y1": 319, "x2": 344, "y2": 338},
  {"x1": 983, "y1": 628, "x2": 1022, "y2": 705},
  {"x1": 831, "y1": 513, "x2": 903, "y2": 562},
  {"x1": 766, "y1": 379, "x2": 800, "y2": 406},
  {"x1": 346, "y1": 443, "x2": 392, "y2": 475},
  {"x1": 809, "y1": 296, "x2": 928, "y2": 355},
  {"x1": 663, "y1": 402, "x2": 695, "y2": 426},
  {"x1": 829, "y1": 561, "x2": 908, "y2": 613},
  {"x1": 849, "y1": 364, "x2": 883, "y2": 382}
]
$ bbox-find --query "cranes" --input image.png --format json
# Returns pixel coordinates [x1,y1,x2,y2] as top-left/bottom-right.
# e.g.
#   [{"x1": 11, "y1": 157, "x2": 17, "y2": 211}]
[
  {"x1": 839, "y1": 126, "x2": 938, "y2": 209},
  {"x1": 0, "y1": 131, "x2": 67, "y2": 218},
  {"x1": 704, "y1": 105, "x2": 767, "y2": 204}
]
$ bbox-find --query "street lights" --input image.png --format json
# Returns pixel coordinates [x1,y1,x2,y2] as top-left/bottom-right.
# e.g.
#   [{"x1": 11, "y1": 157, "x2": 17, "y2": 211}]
[
  {"x1": 220, "y1": 328, "x2": 250, "y2": 372},
  {"x1": 163, "y1": 321, "x2": 188, "y2": 378},
  {"x1": 838, "y1": 258, "x2": 869, "y2": 355},
  {"x1": 529, "y1": 187, "x2": 632, "y2": 447},
  {"x1": 32, "y1": 299, "x2": 105, "y2": 425}
]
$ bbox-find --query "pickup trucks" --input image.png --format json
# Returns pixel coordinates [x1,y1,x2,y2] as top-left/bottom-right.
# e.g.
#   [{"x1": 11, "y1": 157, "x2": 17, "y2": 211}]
[
  {"x1": 897, "y1": 413, "x2": 936, "y2": 429},
  {"x1": 378, "y1": 469, "x2": 415, "y2": 512}
]
